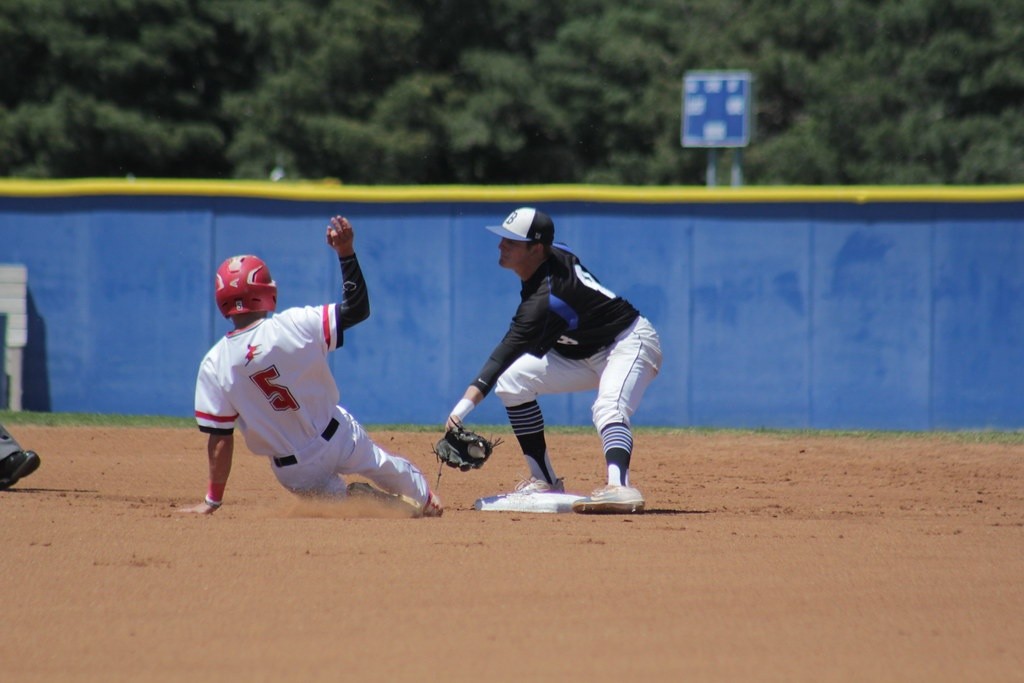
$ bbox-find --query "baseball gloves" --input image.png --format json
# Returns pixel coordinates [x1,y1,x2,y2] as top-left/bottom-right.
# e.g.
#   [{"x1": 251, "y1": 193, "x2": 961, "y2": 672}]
[{"x1": 435, "y1": 427, "x2": 493, "y2": 472}]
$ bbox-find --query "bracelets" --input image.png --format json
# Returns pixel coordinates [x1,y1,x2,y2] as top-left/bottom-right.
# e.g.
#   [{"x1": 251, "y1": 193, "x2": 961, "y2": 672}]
[
  {"x1": 450, "y1": 398, "x2": 475, "y2": 422},
  {"x1": 204, "y1": 480, "x2": 226, "y2": 509}
]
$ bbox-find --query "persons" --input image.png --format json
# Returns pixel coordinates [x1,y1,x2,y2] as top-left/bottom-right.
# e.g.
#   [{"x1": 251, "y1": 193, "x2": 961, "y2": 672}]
[
  {"x1": 0, "y1": 423, "x2": 41, "y2": 490},
  {"x1": 176, "y1": 216, "x2": 442, "y2": 519},
  {"x1": 435, "y1": 206, "x2": 661, "y2": 512}
]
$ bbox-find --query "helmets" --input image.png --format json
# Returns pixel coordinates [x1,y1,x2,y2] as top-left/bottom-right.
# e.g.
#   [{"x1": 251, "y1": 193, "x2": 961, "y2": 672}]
[{"x1": 215, "y1": 256, "x2": 276, "y2": 320}]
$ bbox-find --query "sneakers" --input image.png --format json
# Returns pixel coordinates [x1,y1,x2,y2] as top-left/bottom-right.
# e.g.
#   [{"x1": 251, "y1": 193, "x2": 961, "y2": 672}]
[
  {"x1": 515, "y1": 472, "x2": 565, "y2": 493},
  {"x1": 572, "y1": 485, "x2": 645, "y2": 515},
  {"x1": 423, "y1": 491, "x2": 442, "y2": 516},
  {"x1": 0, "y1": 450, "x2": 41, "y2": 490}
]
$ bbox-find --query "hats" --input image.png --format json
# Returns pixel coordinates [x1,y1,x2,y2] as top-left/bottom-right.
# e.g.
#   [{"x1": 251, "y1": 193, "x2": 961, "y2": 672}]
[{"x1": 486, "y1": 208, "x2": 554, "y2": 245}]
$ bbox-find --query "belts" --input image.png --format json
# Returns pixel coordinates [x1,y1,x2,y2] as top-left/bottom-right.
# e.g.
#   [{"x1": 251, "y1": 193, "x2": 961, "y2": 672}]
[{"x1": 274, "y1": 418, "x2": 340, "y2": 468}]
